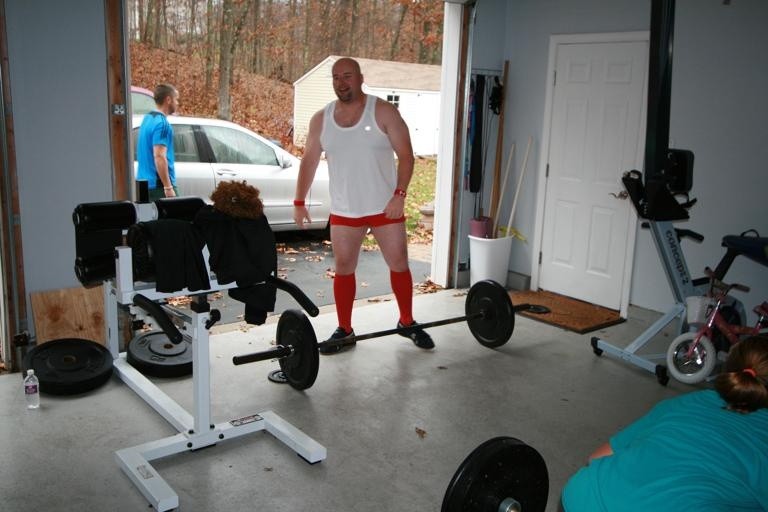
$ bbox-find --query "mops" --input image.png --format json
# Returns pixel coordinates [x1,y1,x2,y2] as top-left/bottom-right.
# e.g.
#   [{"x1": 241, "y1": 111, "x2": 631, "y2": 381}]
[{"x1": 469, "y1": 77, "x2": 493, "y2": 238}]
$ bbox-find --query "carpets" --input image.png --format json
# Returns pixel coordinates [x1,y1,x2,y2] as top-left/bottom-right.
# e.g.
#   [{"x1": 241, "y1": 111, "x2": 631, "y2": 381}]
[{"x1": 506, "y1": 288, "x2": 628, "y2": 336}]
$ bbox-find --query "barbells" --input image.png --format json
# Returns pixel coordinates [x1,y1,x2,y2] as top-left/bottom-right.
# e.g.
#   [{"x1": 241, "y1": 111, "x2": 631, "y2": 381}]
[{"x1": 233, "y1": 279, "x2": 531, "y2": 390}]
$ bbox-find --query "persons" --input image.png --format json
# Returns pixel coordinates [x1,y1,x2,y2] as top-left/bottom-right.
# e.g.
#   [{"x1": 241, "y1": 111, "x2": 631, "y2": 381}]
[
  {"x1": 294, "y1": 59, "x2": 434, "y2": 354},
  {"x1": 557, "y1": 334, "x2": 767, "y2": 511},
  {"x1": 135, "y1": 83, "x2": 179, "y2": 204}
]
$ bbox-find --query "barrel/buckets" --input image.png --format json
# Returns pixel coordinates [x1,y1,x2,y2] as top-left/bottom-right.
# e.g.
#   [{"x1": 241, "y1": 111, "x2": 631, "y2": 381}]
[{"x1": 467, "y1": 227, "x2": 516, "y2": 288}]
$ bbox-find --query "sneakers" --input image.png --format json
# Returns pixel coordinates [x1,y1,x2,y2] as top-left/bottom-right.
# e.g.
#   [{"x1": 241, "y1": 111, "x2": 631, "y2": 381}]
[
  {"x1": 320, "y1": 327, "x2": 357, "y2": 355},
  {"x1": 396, "y1": 320, "x2": 435, "y2": 350}
]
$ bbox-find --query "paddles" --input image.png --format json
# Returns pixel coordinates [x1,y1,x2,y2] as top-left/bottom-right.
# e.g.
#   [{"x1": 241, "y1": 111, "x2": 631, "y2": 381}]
[{"x1": 490, "y1": 60, "x2": 508, "y2": 236}]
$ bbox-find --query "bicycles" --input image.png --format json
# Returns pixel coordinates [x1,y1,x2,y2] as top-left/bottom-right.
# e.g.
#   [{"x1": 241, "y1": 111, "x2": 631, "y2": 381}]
[{"x1": 666, "y1": 266, "x2": 767, "y2": 384}]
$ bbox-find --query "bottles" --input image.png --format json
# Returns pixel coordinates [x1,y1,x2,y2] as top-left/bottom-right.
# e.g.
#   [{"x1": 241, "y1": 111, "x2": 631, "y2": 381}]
[{"x1": 22, "y1": 368, "x2": 40, "y2": 410}]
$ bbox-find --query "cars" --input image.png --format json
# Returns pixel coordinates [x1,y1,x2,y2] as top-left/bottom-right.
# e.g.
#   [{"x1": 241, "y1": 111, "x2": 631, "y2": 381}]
[
  {"x1": 131, "y1": 114, "x2": 331, "y2": 236},
  {"x1": 130, "y1": 85, "x2": 165, "y2": 114}
]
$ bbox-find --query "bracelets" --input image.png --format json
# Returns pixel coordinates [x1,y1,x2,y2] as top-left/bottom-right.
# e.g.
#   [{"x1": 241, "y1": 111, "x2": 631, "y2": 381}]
[
  {"x1": 395, "y1": 189, "x2": 407, "y2": 199},
  {"x1": 163, "y1": 186, "x2": 174, "y2": 190},
  {"x1": 294, "y1": 200, "x2": 305, "y2": 207}
]
